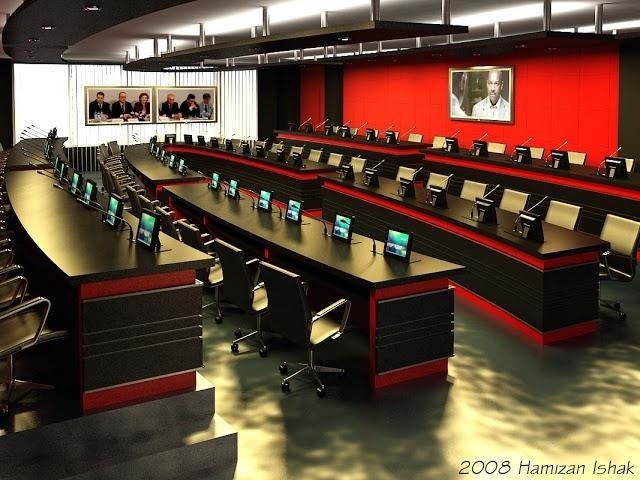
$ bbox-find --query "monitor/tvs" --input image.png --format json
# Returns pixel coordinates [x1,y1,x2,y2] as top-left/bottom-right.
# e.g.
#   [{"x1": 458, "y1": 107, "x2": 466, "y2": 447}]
[
  {"x1": 149, "y1": 124, "x2": 420, "y2": 263},
  {"x1": 44, "y1": 127, "x2": 170, "y2": 253},
  {"x1": 430, "y1": 136, "x2": 628, "y2": 243}
]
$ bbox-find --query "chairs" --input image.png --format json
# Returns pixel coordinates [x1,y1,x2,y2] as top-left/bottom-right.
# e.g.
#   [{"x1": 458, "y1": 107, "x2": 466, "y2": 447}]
[{"x1": 0, "y1": 119, "x2": 640, "y2": 417}]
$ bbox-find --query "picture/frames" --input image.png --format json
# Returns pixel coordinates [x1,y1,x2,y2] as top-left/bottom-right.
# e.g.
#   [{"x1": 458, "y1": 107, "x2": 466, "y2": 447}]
[
  {"x1": 446, "y1": 64, "x2": 516, "y2": 126},
  {"x1": 81, "y1": 85, "x2": 219, "y2": 126}
]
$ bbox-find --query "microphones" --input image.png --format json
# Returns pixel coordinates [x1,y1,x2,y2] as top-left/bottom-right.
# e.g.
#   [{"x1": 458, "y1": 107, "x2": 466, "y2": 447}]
[
  {"x1": 132, "y1": 132, "x2": 377, "y2": 256},
  {"x1": 20, "y1": 124, "x2": 136, "y2": 244},
  {"x1": 294, "y1": 117, "x2": 622, "y2": 238}
]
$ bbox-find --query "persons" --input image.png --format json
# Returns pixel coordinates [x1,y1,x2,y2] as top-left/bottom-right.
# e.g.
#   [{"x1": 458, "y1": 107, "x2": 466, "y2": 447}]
[
  {"x1": 452, "y1": 72, "x2": 467, "y2": 118},
  {"x1": 198, "y1": 93, "x2": 214, "y2": 118},
  {"x1": 133, "y1": 93, "x2": 150, "y2": 114},
  {"x1": 89, "y1": 92, "x2": 111, "y2": 120},
  {"x1": 112, "y1": 92, "x2": 134, "y2": 120},
  {"x1": 180, "y1": 94, "x2": 200, "y2": 118},
  {"x1": 472, "y1": 70, "x2": 509, "y2": 120},
  {"x1": 162, "y1": 94, "x2": 180, "y2": 119}
]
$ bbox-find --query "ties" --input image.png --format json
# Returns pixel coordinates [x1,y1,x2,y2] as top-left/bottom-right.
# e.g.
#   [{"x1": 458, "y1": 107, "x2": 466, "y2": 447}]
[
  {"x1": 205, "y1": 104, "x2": 207, "y2": 112},
  {"x1": 121, "y1": 104, "x2": 124, "y2": 112}
]
